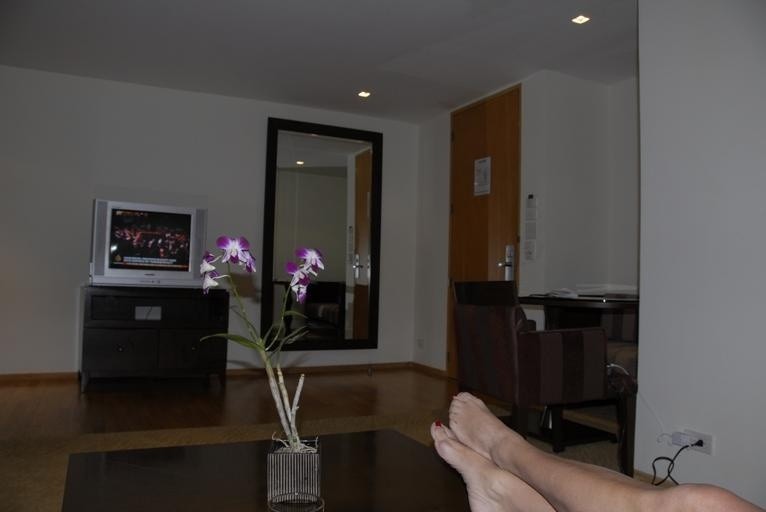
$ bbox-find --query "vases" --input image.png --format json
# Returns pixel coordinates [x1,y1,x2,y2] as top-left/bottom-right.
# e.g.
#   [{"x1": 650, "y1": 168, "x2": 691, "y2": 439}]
[{"x1": 267, "y1": 438, "x2": 326, "y2": 506}]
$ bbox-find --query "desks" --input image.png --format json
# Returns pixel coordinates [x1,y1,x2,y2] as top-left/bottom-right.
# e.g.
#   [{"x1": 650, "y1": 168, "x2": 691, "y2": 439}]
[
  {"x1": 74, "y1": 286, "x2": 230, "y2": 400},
  {"x1": 520, "y1": 280, "x2": 636, "y2": 446},
  {"x1": 57, "y1": 427, "x2": 482, "y2": 511}
]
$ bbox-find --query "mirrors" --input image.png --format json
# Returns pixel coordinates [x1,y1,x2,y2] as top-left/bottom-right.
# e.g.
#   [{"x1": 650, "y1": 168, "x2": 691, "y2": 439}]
[{"x1": 260, "y1": 118, "x2": 381, "y2": 349}]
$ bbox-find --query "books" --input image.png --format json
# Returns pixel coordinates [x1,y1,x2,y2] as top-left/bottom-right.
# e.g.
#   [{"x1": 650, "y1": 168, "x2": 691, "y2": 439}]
[{"x1": 530, "y1": 282, "x2": 637, "y2": 301}]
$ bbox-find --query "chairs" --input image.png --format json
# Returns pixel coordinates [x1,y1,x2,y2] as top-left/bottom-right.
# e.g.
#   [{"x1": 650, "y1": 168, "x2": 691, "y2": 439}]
[{"x1": 454, "y1": 276, "x2": 634, "y2": 454}]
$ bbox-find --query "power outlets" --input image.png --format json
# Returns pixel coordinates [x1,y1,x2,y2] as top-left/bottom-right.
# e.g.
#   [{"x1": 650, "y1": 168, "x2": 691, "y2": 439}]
[{"x1": 672, "y1": 429, "x2": 714, "y2": 458}]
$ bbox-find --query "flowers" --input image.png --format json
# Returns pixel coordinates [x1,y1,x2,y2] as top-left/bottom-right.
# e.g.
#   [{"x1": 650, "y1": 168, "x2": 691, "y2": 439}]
[{"x1": 196, "y1": 237, "x2": 326, "y2": 447}]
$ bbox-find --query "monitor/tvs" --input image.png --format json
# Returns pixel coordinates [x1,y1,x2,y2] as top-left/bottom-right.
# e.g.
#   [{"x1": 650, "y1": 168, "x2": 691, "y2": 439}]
[{"x1": 89, "y1": 198, "x2": 207, "y2": 288}]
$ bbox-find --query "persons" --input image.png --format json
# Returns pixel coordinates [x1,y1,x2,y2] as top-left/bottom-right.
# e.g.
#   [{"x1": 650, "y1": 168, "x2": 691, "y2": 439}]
[{"x1": 430, "y1": 390, "x2": 765, "y2": 512}]
